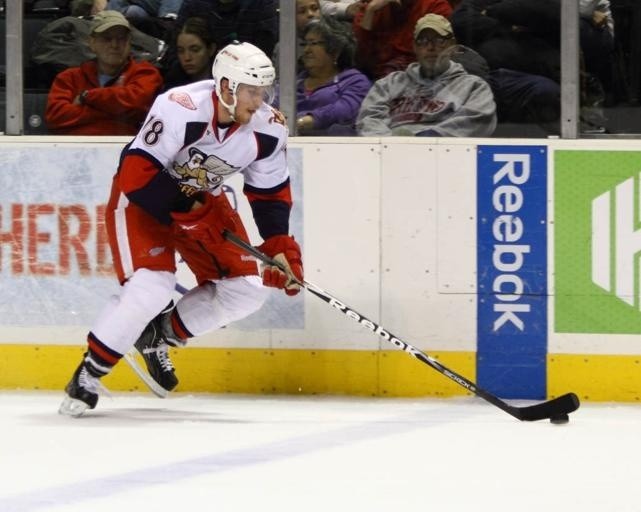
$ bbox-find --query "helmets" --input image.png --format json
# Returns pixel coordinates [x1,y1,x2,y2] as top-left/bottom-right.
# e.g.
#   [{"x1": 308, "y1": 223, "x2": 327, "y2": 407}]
[{"x1": 211, "y1": 40, "x2": 276, "y2": 96}]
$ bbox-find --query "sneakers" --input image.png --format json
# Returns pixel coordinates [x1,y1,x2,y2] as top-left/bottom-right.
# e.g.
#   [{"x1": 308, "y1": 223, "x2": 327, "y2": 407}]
[
  {"x1": 134, "y1": 313, "x2": 180, "y2": 392},
  {"x1": 63, "y1": 351, "x2": 100, "y2": 409}
]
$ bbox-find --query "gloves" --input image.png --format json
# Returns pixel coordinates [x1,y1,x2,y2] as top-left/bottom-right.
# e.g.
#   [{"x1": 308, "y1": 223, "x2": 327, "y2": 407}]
[
  {"x1": 168, "y1": 190, "x2": 238, "y2": 254},
  {"x1": 256, "y1": 233, "x2": 305, "y2": 297}
]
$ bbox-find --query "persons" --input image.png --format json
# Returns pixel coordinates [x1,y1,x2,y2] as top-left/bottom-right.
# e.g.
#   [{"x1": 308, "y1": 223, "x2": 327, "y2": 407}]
[
  {"x1": 65, "y1": 40, "x2": 303, "y2": 409},
  {"x1": 2, "y1": 0, "x2": 641, "y2": 137}
]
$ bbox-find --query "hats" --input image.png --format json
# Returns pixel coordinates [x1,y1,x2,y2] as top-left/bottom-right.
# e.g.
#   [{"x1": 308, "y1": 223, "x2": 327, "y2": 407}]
[
  {"x1": 413, "y1": 12, "x2": 454, "y2": 39},
  {"x1": 87, "y1": 9, "x2": 131, "y2": 36}
]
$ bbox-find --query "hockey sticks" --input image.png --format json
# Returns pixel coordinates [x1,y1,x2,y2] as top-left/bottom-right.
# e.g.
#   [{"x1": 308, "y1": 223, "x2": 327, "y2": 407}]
[{"x1": 223, "y1": 230, "x2": 579, "y2": 421}]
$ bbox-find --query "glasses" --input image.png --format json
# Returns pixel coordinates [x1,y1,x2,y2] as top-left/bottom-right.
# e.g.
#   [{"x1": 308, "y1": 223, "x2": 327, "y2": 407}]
[{"x1": 416, "y1": 35, "x2": 452, "y2": 48}]
